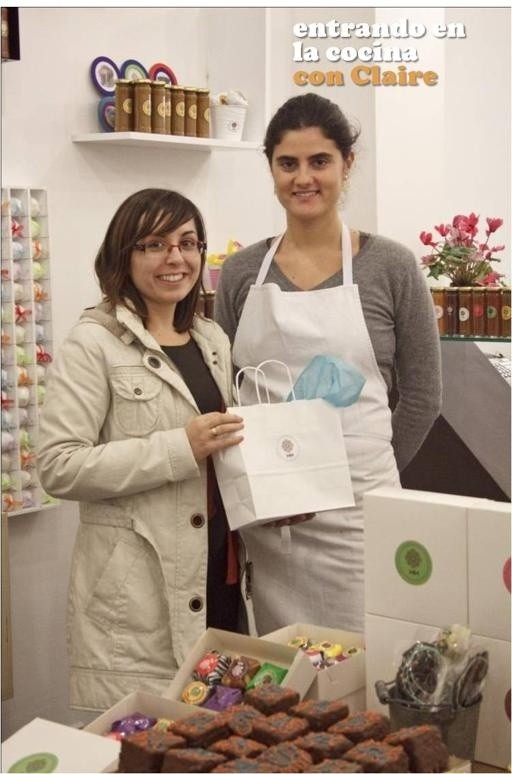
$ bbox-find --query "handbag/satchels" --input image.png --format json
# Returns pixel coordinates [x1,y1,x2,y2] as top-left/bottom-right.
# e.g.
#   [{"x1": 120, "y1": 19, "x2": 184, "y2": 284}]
[{"x1": 212, "y1": 359, "x2": 357, "y2": 532}]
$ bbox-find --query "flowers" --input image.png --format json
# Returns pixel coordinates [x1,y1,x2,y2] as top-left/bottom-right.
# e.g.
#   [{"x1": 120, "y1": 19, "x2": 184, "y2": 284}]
[{"x1": 420, "y1": 212, "x2": 505, "y2": 287}]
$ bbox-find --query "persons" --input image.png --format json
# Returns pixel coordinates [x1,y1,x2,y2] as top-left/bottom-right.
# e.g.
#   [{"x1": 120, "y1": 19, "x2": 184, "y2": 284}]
[
  {"x1": 36, "y1": 188, "x2": 316, "y2": 713},
  {"x1": 214, "y1": 92, "x2": 444, "y2": 638}
]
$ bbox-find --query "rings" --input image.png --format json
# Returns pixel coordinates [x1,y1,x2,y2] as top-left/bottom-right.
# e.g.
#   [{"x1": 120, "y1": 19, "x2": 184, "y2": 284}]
[{"x1": 212, "y1": 428, "x2": 217, "y2": 436}]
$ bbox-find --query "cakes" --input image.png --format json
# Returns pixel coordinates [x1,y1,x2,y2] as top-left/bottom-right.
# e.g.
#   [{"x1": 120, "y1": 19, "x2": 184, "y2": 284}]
[{"x1": 112, "y1": 634, "x2": 447, "y2": 773}]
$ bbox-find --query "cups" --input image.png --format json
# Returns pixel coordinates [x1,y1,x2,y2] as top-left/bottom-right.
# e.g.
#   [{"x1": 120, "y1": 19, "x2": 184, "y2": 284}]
[{"x1": 211, "y1": 105, "x2": 247, "y2": 142}]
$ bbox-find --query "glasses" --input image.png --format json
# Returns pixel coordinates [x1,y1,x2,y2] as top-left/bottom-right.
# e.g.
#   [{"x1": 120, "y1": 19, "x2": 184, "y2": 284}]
[{"x1": 134, "y1": 238, "x2": 205, "y2": 256}]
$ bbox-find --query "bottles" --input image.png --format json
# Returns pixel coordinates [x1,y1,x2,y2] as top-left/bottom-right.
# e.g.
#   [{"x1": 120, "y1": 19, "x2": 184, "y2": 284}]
[
  {"x1": 194, "y1": 292, "x2": 216, "y2": 319},
  {"x1": 429, "y1": 286, "x2": 511, "y2": 340},
  {"x1": 113, "y1": 78, "x2": 211, "y2": 140}
]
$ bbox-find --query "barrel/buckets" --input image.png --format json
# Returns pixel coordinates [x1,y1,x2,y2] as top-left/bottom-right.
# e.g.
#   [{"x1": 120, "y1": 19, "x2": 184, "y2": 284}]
[{"x1": 374, "y1": 677, "x2": 480, "y2": 769}]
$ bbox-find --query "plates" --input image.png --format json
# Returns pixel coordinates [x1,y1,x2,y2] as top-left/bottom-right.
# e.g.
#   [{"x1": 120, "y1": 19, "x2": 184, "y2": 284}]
[{"x1": 90, "y1": 57, "x2": 179, "y2": 131}]
